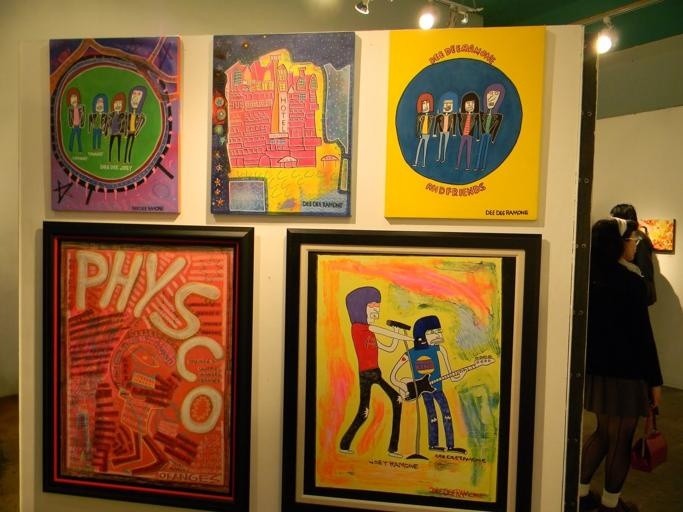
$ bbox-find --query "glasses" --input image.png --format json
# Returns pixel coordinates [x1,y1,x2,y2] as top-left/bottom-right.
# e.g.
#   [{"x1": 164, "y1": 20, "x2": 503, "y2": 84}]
[{"x1": 625, "y1": 236, "x2": 642, "y2": 245}]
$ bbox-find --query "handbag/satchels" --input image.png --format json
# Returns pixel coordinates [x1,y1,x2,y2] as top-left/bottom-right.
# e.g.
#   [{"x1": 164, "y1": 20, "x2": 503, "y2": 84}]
[{"x1": 631, "y1": 431, "x2": 667, "y2": 474}]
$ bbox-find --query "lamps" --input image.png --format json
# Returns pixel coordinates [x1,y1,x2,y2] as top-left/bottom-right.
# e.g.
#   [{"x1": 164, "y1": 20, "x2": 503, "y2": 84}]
[
  {"x1": 597, "y1": 16, "x2": 613, "y2": 54},
  {"x1": 458, "y1": 6, "x2": 469, "y2": 24},
  {"x1": 355, "y1": 0, "x2": 369, "y2": 14},
  {"x1": 418, "y1": 0, "x2": 435, "y2": 30}
]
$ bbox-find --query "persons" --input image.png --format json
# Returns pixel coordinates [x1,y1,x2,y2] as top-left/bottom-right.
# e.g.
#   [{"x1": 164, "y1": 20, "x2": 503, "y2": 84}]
[
  {"x1": 578, "y1": 218, "x2": 663, "y2": 512},
  {"x1": 411, "y1": 83, "x2": 505, "y2": 171},
  {"x1": 611, "y1": 203, "x2": 658, "y2": 308},
  {"x1": 65, "y1": 85, "x2": 147, "y2": 164},
  {"x1": 339, "y1": 286, "x2": 468, "y2": 458}
]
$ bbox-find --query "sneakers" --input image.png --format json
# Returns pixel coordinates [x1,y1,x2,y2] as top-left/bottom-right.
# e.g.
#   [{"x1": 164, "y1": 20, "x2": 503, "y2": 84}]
[
  {"x1": 580, "y1": 491, "x2": 600, "y2": 512},
  {"x1": 600, "y1": 498, "x2": 637, "y2": 511}
]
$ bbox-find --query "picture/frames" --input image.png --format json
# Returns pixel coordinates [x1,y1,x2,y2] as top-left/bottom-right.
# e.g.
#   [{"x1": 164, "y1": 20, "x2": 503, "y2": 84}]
[
  {"x1": 282, "y1": 228, "x2": 542, "y2": 512},
  {"x1": 43, "y1": 221, "x2": 254, "y2": 512}
]
